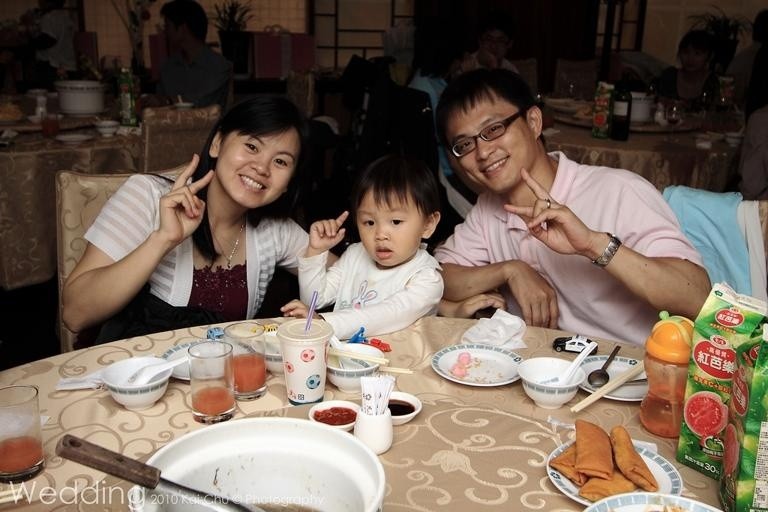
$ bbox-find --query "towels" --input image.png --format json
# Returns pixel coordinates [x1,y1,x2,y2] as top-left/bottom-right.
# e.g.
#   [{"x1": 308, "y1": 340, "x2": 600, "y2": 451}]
[{"x1": 459, "y1": 305, "x2": 529, "y2": 354}]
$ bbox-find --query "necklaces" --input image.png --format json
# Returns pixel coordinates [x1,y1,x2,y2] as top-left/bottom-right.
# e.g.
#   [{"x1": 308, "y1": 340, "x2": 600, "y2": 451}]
[{"x1": 207, "y1": 219, "x2": 244, "y2": 269}]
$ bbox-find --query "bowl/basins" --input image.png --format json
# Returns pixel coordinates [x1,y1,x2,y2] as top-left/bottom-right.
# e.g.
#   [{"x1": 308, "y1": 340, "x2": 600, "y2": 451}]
[
  {"x1": 326, "y1": 343, "x2": 386, "y2": 391},
  {"x1": 134, "y1": 416, "x2": 385, "y2": 511},
  {"x1": 105, "y1": 356, "x2": 174, "y2": 411},
  {"x1": 516, "y1": 357, "x2": 586, "y2": 409},
  {"x1": 95, "y1": 121, "x2": 119, "y2": 138}
]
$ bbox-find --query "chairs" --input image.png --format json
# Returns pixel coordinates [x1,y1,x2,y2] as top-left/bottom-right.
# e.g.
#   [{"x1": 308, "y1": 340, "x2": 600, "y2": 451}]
[
  {"x1": 643, "y1": 184, "x2": 765, "y2": 319},
  {"x1": 47, "y1": 157, "x2": 209, "y2": 352},
  {"x1": 141, "y1": 53, "x2": 766, "y2": 214}
]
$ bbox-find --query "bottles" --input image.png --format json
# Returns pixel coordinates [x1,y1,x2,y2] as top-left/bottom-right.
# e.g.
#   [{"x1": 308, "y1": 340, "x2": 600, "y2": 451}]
[
  {"x1": 637, "y1": 311, "x2": 696, "y2": 440},
  {"x1": 612, "y1": 70, "x2": 633, "y2": 142},
  {"x1": 116, "y1": 71, "x2": 138, "y2": 127},
  {"x1": 34, "y1": 94, "x2": 48, "y2": 119},
  {"x1": 591, "y1": 83, "x2": 612, "y2": 139}
]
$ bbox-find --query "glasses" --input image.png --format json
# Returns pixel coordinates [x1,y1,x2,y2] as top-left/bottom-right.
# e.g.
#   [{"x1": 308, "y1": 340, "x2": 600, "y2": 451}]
[{"x1": 446, "y1": 111, "x2": 523, "y2": 159}]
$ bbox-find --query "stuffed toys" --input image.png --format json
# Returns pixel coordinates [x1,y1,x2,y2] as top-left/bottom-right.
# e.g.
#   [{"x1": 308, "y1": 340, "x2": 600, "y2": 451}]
[
  {"x1": 348, "y1": 326, "x2": 367, "y2": 343},
  {"x1": 257, "y1": 321, "x2": 278, "y2": 333},
  {"x1": 550, "y1": 336, "x2": 598, "y2": 356},
  {"x1": 367, "y1": 339, "x2": 392, "y2": 353},
  {"x1": 206, "y1": 325, "x2": 225, "y2": 342}
]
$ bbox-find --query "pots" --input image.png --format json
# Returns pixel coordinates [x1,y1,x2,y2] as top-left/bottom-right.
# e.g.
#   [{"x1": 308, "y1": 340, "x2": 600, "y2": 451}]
[{"x1": 54, "y1": 79, "x2": 108, "y2": 118}]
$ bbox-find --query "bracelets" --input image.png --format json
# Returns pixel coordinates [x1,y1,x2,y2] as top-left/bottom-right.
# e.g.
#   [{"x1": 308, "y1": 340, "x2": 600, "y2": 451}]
[
  {"x1": 592, "y1": 232, "x2": 621, "y2": 267},
  {"x1": 167, "y1": 96, "x2": 174, "y2": 105}
]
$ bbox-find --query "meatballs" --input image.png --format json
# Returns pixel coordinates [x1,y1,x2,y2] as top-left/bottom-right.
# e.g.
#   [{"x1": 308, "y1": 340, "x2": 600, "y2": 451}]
[
  {"x1": 453, "y1": 364, "x2": 465, "y2": 378},
  {"x1": 458, "y1": 350, "x2": 471, "y2": 364}
]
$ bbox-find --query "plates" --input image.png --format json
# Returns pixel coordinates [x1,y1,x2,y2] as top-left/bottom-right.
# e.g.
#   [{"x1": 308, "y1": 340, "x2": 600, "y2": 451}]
[
  {"x1": 546, "y1": 440, "x2": 682, "y2": 505},
  {"x1": 165, "y1": 340, "x2": 253, "y2": 380},
  {"x1": 307, "y1": 399, "x2": 360, "y2": 432},
  {"x1": 581, "y1": 492, "x2": 724, "y2": 511},
  {"x1": 544, "y1": 98, "x2": 583, "y2": 114},
  {"x1": 387, "y1": 389, "x2": 423, "y2": 426},
  {"x1": 54, "y1": 132, "x2": 95, "y2": 146},
  {"x1": 47, "y1": 113, "x2": 64, "y2": 121},
  {"x1": 576, "y1": 354, "x2": 649, "y2": 402},
  {"x1": 28, "y1": 115, "x2": 43, "y2": 124},
  {"x1": 431, "y1": 343, "x2": 526, "y2": 388}
]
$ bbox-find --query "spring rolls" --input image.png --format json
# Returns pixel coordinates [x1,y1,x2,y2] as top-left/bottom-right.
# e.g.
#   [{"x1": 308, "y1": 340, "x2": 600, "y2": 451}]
[{"x1": 549, "y1": 418, "x2": 658, "y2": 503}]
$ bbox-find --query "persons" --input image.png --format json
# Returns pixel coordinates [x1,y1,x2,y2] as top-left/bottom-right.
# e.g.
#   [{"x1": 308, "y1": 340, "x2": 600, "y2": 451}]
[
  {"x1": 433, "y1": 69, "x2": 712, "y2": 347},
  {"x1": 408, "y1": 42, "x2": 463, "y2": 177},
  {"x1": 12, "y1": 0, "x2": 83, "y2": 93},
  {"x1": 62, "y1": 94, "x2": 506, "y2": 350},
  {"x1": 567, "y1": 9, "x2": 768, "y2": 199},
  {"x1": 279, "y1": 154, "x2": 445, "y2": 342},
  {"x1": 141, "y1": 0, "x2": 230, "y2": 108},
  {"x1": 449, "y1": 28, "x2": 522, "y2": 75}
]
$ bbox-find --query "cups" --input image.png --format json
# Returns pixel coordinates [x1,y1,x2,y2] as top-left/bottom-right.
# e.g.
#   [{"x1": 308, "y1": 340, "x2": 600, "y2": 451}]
[
  {"x1": 0, "y1": 383, "x2": 44, "y2": 484},
  {"x1": 188, "y1": 319, "x2": 333, "y2": 424},
  {"x1": 355, "y1": 407, "x2": 394, "y2": 455},
  {"x1": 176, "y1": 103, "x2": 193, "y2": 110},
  {"x1": 628, "y1": 90, "x2": 745, "y2": 151}
]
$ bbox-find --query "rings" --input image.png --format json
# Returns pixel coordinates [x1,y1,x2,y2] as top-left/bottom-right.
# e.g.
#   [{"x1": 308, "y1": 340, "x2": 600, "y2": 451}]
[{"x1": 544, "y1": 198, "x2": 551, "y2": 210}]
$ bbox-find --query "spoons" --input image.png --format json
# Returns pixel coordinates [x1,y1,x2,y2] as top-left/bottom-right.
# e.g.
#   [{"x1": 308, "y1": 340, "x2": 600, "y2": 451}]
[
  {"x1": 127, "y1": 352, "x2": 200, "y2": 387},
  {"x1": 540, "y1": 341, "x2": 598, "y2": 386},
  {"x1": 588, "y1": 345, "x2": 621, "y2": 388},
  {"x1": 329, "y1": 333, "x2": 369, "y2": 371}
]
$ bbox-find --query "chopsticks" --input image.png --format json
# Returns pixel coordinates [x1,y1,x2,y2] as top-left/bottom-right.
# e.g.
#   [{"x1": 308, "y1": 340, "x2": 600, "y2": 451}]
[
  {"x1": 570, "y1": 360, "x2": 645, "y2": 414},
  {"x1": 325, "y1": 348, "x2": 390, "y2": 367},
  {"x1": 378, "y1": 366, "x2": 413, "y2": 374}
]
